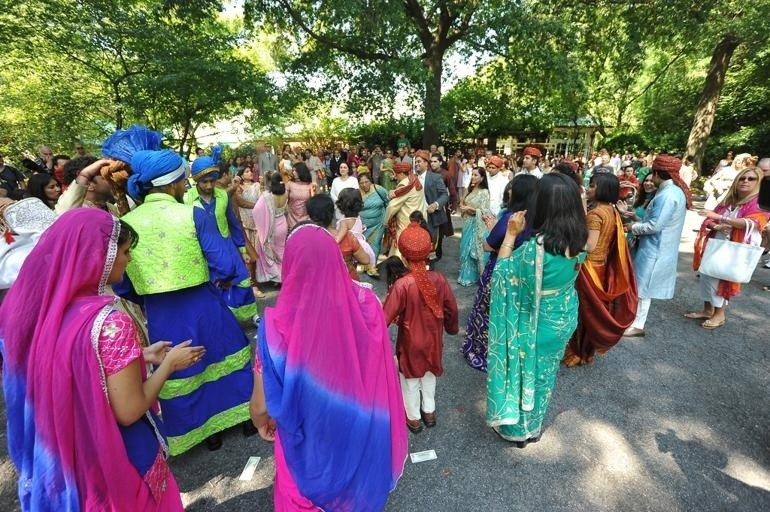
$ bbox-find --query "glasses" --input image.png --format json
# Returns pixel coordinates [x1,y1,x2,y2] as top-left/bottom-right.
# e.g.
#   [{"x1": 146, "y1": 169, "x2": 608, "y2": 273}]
[
  {"x1": 76, "y1": 147, "x2": 83, "y2": 150},
  {"x1": 739, "y1": 176, "x2": 757, "y2": 181}
]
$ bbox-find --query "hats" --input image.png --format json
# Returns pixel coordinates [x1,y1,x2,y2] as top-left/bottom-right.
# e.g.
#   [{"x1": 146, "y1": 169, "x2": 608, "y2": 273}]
[
  {"x1": 523, "y1": 146, "x2": 542, "y2": 158},
  {"x1": 397, "y1": 226, "x2": 433, "y2": 261},
  {"x1": 191, "y1": 156, "x2": 219, "y2": 181},
  {"x1": 488, "y1": 156, "x2": 503, "y2": 168},
  {"x1": 392, "y1": 161, "x2": 413, "y2": 174},
  {"x1": 652, "y1": 153, "x2": 682, "y2": 173},
  {"x1": 130, "y1": 148, "x2": 186, "y2": 188},
  {"x1": 414, "y1": 149, "x2": 431, "y2": 162}
]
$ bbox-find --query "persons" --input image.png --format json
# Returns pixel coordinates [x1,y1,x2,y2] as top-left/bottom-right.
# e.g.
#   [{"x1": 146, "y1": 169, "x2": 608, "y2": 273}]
[
  {"x1": 1, "y1": 126, "x2": 697, "y2": 373},
  {"x1": 485, "y1": 173, "x2": 589, "y2": 442},
  {"x1": 249, "y1": 221, "x2": 408, "y2": 511},
  {"x1": 111, "y1": 148, "x2": 253, "y2": 457},
  {"x1": 703, "y1": 150, "x2": 770, "y2": 269},
  {"x1": 685, "y1": 167, "x2": 769, "y2": 330},
  {"x1": 381, "y1": 226, "x2": 459, "y2": 434},
  {"x1": 0, "y1": 208, "x2": 206, "y2": 512}
]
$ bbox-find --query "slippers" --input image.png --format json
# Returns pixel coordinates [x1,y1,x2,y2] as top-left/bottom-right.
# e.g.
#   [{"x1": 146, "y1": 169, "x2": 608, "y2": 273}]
[
  {"x1": 702, "y1": 316, "x2": 727, "y2": 330},
  {"x1": 687, "y1": 310, "x2": 713, "y2": 319}
]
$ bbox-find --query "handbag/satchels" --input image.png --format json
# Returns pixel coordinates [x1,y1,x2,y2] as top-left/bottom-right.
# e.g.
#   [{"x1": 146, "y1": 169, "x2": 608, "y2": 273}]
[{"x1": 697, "y1": 237, "x2": 766, "y2": 284}]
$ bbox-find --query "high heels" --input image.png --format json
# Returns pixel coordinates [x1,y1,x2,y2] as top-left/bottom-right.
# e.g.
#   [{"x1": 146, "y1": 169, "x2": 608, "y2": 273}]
[{"x1": 492, "y1": 426, "x2": 525, "y2": 448}]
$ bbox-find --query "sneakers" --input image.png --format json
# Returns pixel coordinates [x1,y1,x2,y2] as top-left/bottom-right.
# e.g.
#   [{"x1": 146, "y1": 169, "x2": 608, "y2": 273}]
[
  {"x1": 406, "y1": 417, "x2": 423, "y2": 434},
  {"x1": 421, "y1": 410, "x2": 437, "y2": 427}
]
